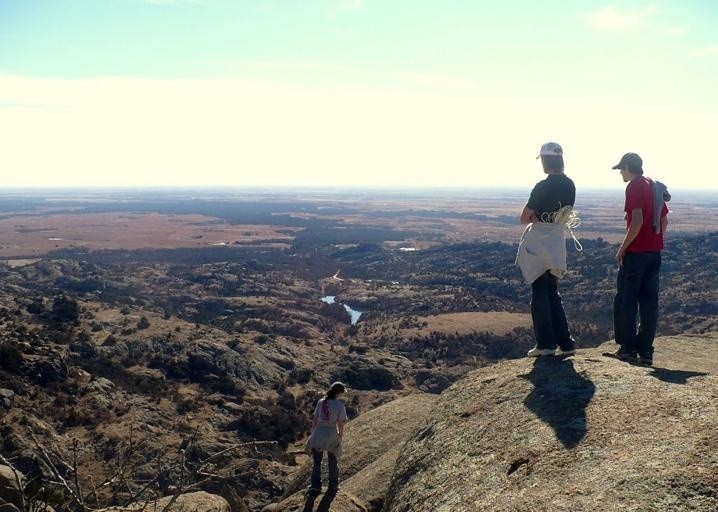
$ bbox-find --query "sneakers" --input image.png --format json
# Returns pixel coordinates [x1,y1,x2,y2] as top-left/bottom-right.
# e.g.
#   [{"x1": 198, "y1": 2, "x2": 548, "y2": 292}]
[
  {"x1": 311, "y1": 484, "x2": 340, "y2": 491},
  {"x1": 554, "y1": 345, "x2": 577, "y2": 356},
  {"x1": 527, "y1": 345, "x2": 554, "y2": 357},
  {"x1": 602, "y1": 345, "x2": 653, "y2": 366}
]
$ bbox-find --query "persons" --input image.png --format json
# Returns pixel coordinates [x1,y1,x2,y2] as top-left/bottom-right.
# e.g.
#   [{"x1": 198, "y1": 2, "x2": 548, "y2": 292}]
[
  {"x1": 612, "y1": 153, "x2": 668, "y2": 365},
  {"x1": 305, "y1": 382, "x2": 348, "y2": 490},
  {"x1": 520, "y1": 142, "x2": 576, "y2": 356}
]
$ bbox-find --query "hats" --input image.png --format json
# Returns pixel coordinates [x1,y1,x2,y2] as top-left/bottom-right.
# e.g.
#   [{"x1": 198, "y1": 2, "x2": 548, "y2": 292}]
[
  {"x1": 613, "y1": 153, "x2": 643, "y2": 171},
  {"x1": 536, "y1": 142, "x2": 562, "y2": 160},
  {"x1": 332, "y1": 383, "x2": 345, "y2": 393}
]
